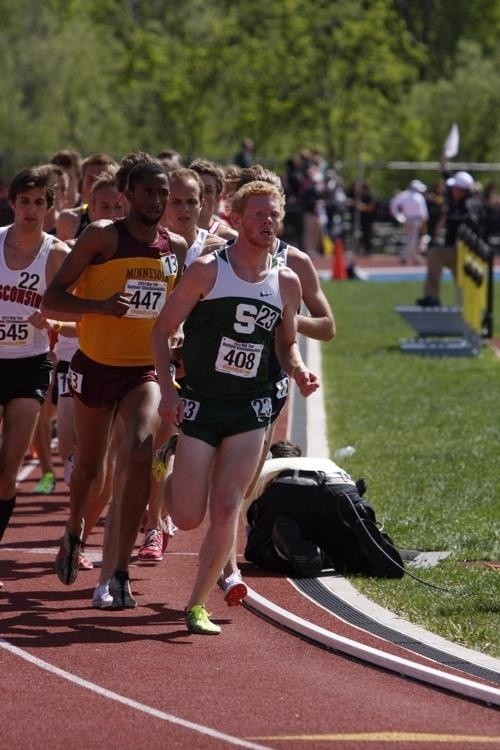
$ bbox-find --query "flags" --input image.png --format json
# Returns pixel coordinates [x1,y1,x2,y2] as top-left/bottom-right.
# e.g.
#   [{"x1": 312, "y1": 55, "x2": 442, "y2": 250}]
[{"x1": 443, "y1": 121, "x2": 461, "y2": 159}]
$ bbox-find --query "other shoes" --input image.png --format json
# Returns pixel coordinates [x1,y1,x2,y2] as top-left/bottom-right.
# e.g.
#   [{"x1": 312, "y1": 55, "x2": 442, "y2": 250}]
[
  {"x1": 64, "y1": 453, "x2": 76, "y2": 487},
  {"x1": 167, "y1": 516, "x2": 179, "y2": 538}
]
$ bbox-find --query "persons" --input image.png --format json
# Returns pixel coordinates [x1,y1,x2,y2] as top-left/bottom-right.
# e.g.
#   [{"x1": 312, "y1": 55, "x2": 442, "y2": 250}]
[
  {"x1": 338, "y1": 152, "x2": 500, "y2": 314},
  {"x1": 2, "y1": 136, "x2": 338, "y2": 634}
]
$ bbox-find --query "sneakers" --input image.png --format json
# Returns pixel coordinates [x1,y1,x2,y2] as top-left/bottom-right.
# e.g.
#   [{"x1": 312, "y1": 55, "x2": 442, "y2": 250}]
[
  {"x1": 31, "y1": 471, "x2": 56, "y2": 496},
  {"x1": 217, "y1": 571, "x2": 246, "y2": 608},
  {"x1": 79, "y1": 552, "x2": 95, "y2": 573},
  {"x1": 183, "y1": 604, "x2": 220, "y2": 633},
  {"x1": 54, "y1": 532, "x2": 84, "y2": 586},
  {"x1": 91, "y1": 581, "x2": 114, "y2": 609},
  {"x1": 110, "y1": 572, "x2": 137, "y2": 608},
  {"x1": 150, "y1": 434, "x2": 179, "y2": 484},
  {"x1": 272, "y1": 514, "x2": 322, "y2": 578},
  {"x1": 136, "y1": 529, "x2": 165, "y2": 564},
  {"x1": 352, "y1": 517, "x2": 404, "y2": 579}
]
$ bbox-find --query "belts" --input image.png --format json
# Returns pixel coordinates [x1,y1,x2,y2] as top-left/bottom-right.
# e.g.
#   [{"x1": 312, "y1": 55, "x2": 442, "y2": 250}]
[{"x1": 276, "y1": 470, "x2": 350, "y2": 479}]
